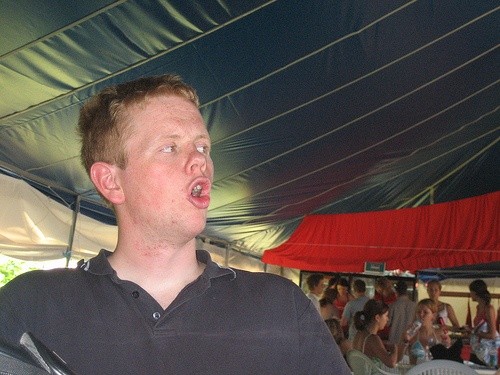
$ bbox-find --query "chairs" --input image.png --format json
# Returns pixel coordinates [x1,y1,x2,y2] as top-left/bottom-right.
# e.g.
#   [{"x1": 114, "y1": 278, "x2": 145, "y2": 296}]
[{"x1": 346, "y1": 347, "x2": 480, "y2": 375}]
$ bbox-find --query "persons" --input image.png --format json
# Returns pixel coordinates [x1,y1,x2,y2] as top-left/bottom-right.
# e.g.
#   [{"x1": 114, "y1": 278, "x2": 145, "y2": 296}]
[
  {"x1": 387, "y1": 282, "x2": 417, "y2": 345},
  {"x1": 426, "y1": 280, "x2": 461, "y2": 329},
  {"x1": 467, "y1": 279, "x2": 500, "y2": 368},
  {"x1": 396, "y1": 298, "x2": 445, "y2": 365},
  {"x1": 305, "y1": 273, "x2": 324, "y2": 316},
  {"x1": 324, "y1": 318, "x2": 354, "y2": 358},
  {"x1": 374, "y1": 276, "x2": 398, "y2": 341},
  {"x1": 0, "y1": 72, "x2": 353, "y2": 375},
  {"x1": 353, "y1": 296, "x2": 401, "y2": 368},
  {"x1": 318, "y1": 275, "x2": 372, "y2": 341}
]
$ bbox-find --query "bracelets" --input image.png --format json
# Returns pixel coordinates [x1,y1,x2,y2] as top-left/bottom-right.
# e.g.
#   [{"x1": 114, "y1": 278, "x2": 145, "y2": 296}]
[{"x1": 400, "y1": 339, "x2": 409, "y2": 346}]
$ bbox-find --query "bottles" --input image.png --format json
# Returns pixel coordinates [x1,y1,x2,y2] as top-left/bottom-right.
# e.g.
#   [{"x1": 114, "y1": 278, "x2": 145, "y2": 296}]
[{"x1": 406, "y1": 320, "x2": 423, "y2": 337}]
